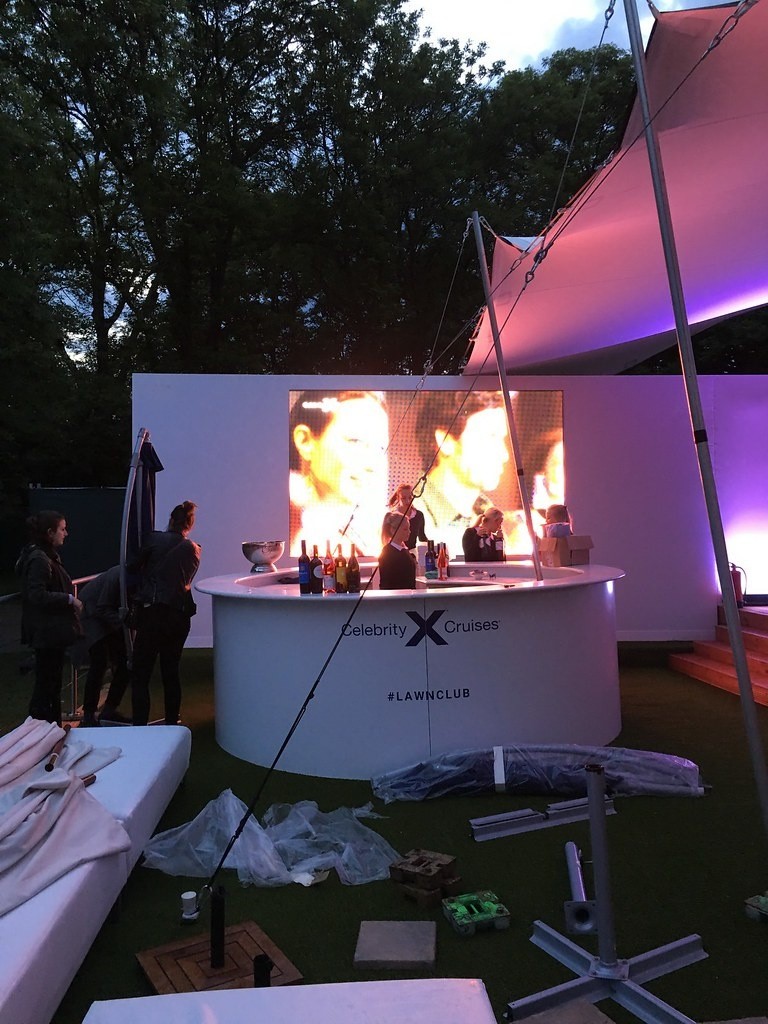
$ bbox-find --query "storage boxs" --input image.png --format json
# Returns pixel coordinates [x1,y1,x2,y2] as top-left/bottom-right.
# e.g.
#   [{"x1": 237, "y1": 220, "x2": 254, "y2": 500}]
[{"x1": 539, "y1": 536, "x2": 595, "y2": 566}]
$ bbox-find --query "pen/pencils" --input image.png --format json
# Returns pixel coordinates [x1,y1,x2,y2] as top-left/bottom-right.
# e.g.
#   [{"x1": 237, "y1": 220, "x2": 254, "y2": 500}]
[
  {"x1": 44, "y1": 723, "x2": 71, "y2": 772},
  {"x1": 82, "y1": 774, "x2": 97, "y2": 787}
]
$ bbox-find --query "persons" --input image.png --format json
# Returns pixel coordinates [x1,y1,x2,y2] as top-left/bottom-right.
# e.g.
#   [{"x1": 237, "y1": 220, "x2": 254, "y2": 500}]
[
  {"x1": 413, "y1": 391, "x2": 509, "y2": 558},
  {"x1": 77, "y1": 550, "x2": 145, "y2": 728},
  {"x1": 128, "y1": 501, "x2": 202, "y2": 726},
  {"x1": 15, "y1": 510, "x2": 84, "y2": 727},
  {"x1": 378, "y1": 512, "x2": 416, "y2": 590},
  {"x1": 546, "y1": 504, "x2": 573, "y2": 535},
  {"x1": 461, "y1": 507, "x2": 507, "y2": 561},
  {"x1": 386, "y1": 483, "x2": 428, "y2": 550},
  {"x1": 501, "y1": 429, "x2": 565, "y2": 555},
  {"x1": 289, "y1": 390, "x2": 389, "y2": 556}
]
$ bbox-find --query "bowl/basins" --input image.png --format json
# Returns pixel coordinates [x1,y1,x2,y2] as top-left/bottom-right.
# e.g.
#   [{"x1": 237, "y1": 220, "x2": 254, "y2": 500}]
[{"x1": 241, "y1": 540, "x2": 286, "y2": 572}]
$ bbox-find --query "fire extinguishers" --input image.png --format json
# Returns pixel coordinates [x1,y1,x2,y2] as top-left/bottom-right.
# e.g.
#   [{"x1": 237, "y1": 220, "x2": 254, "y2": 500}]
[{"x1": 728, "y1": 561, "x2": 748, "y2": 609}]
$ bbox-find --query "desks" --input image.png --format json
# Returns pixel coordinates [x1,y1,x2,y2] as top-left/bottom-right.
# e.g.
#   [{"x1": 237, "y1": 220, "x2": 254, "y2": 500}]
[{"x1": 82, "y1": 977, "x2": 498, "y2": 1024}]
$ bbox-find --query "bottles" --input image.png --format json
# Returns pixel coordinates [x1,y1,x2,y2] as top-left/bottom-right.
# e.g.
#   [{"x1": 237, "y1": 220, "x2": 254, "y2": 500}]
[
  {"x1": 425, "y1": 540, "x2": 450, "y2": 580},
  {"x1": 322, "y1": 540, "x2": 336, "y2": 594},
  {"x1": 335, "y1": 544, "x2": 348, "y2": 593},
  {"x1": 347, "y1": 544, "x2": 361, "y2": 593},
  {"x1": 309, "y1": 545, "x2": 323, "y2": 594},
  {"x1": 298, "y1": 540, "x2": 310, "y2": 594}
]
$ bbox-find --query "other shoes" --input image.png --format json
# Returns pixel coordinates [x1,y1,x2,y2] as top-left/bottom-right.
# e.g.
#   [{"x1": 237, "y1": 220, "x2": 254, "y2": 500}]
[{"x1": 100, "y1": 712, "x2": 131, "y2": 723}]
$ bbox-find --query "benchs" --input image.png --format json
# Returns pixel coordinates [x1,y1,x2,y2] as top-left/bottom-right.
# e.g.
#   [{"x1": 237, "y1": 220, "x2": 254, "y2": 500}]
[{"x1": 0, "y1": 725, "x2": 194, "y2": 1024}]
